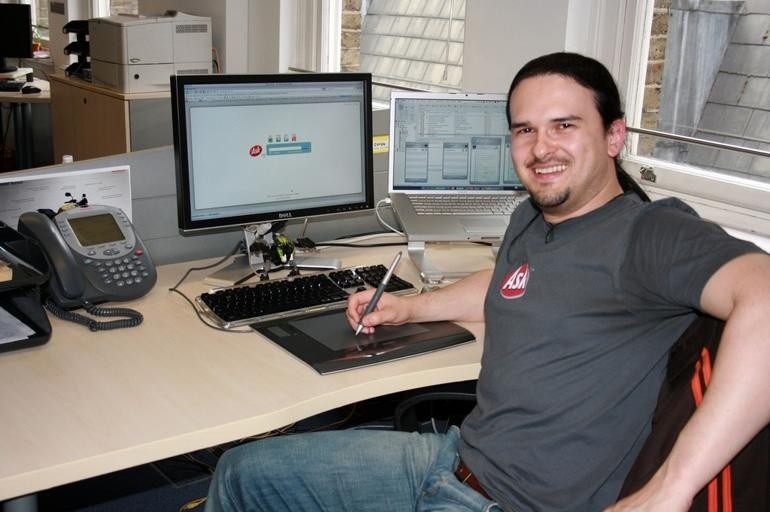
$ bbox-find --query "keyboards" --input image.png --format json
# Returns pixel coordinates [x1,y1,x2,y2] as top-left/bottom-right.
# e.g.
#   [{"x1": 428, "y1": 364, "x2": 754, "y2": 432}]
[
  {"x1": 195, "y1": 262, "x2": 418, "y2": 328},
  {"x1": 0, "y1": 78, "x2": 26, "y2": 92}
]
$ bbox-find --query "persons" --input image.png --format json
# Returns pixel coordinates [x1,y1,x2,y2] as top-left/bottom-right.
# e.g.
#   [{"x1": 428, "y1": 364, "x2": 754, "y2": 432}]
[{"x1": 200, "y1": 50, "x2": 769, "y2": 512}]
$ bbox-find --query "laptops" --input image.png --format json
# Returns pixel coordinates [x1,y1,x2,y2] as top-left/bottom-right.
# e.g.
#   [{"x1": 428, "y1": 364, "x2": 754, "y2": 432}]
[{"x1": 388, "y1": 91, "x2": 531, "y2": 244}]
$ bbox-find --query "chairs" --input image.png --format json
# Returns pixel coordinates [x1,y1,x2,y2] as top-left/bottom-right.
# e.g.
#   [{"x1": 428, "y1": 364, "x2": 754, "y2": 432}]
[{"x1": 394, "y1": 331, "x2": 733, "y2": 512}]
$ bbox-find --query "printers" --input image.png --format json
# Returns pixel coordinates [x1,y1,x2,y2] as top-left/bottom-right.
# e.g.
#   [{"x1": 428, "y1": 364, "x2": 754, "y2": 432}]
[{"x1": 88, "y1": 8, "x2": 214, "y2": 93}]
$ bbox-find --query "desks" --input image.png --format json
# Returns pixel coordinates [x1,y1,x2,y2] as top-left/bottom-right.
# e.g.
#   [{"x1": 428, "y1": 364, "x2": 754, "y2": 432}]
[
  {"x1": 0, "y1": 76, "x2": 51, "y2": 170},
  {"x1": 0, "y1": 232, "x2": 497, "y2": 512}
]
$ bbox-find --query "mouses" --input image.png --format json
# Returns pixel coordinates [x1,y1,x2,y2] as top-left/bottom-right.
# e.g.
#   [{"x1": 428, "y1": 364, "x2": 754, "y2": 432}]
[{"x1": 21, "y1": 85, "x2": 42, "y2": 94}]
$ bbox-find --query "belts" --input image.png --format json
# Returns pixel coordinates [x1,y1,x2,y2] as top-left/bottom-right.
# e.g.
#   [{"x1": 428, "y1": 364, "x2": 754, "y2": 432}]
[{"x1": 445, "y1": 444, "x2": 497, "y2": 504}]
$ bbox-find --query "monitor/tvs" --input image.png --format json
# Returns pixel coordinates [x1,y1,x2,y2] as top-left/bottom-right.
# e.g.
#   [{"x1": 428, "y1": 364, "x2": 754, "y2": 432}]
[
  {"x1": 169, "y1": 71, "x2": 375, "y2": 286},
  {"x1": 0, "y1": 3, "x2": 33, "y2": 72}
]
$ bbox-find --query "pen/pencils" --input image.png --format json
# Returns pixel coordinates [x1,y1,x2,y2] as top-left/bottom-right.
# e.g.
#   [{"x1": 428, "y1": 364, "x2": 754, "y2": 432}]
[{"x1": 354, "y1": 250, "x2": 403, "y2": 337}]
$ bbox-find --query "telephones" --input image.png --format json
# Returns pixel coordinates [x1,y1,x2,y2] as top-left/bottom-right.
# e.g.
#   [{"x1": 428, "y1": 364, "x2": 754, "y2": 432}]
[{"x1": 19, "y1": 204, "x2": 157, "y2": 309}]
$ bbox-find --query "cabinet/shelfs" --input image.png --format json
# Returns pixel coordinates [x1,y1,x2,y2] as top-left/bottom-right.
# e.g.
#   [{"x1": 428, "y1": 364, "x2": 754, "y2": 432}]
[{"x1": 48, "y1": 73, "x2": 174, "y2": 163}]
